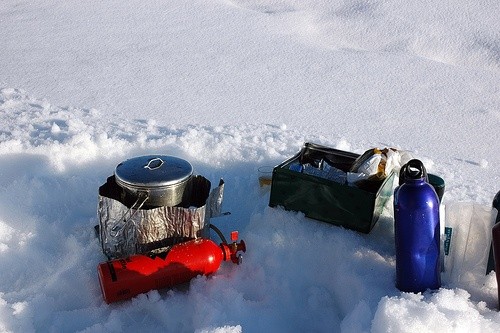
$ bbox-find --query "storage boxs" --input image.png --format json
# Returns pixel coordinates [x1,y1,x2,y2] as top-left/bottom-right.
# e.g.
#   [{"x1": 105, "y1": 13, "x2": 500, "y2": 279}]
[{"x1": 269, "y1": 142, "x2": 395, "y2": 233}]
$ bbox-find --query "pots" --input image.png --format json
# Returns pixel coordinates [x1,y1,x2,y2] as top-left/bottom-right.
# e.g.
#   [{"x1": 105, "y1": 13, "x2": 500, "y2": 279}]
[{"x1": 110, "y1": 154, "x2": 195, "y2": 236}]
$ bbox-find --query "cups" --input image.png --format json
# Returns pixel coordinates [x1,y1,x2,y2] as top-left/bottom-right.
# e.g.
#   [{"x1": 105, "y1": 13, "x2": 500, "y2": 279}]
[{"x1": 258, "y1": 165, "x2": 274, "y2": 187}]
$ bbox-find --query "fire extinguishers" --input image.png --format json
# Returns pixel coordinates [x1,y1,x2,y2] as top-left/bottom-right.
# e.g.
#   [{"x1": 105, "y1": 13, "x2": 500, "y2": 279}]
[{"x1": 97, "y1": 230, "x2": 246, "y2": 305}]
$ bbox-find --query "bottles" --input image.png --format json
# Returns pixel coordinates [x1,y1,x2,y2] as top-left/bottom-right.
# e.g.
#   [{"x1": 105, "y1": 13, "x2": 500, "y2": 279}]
[{"x1": 393, "y1": 158, "x2": 441, "y2": 291}]
